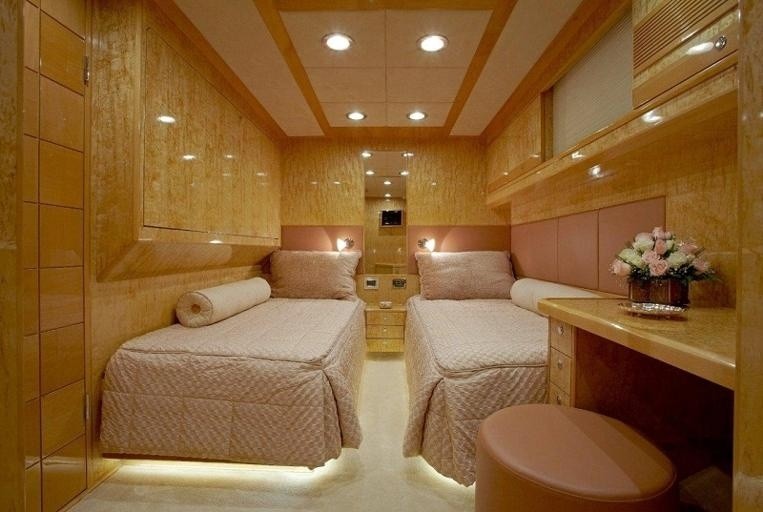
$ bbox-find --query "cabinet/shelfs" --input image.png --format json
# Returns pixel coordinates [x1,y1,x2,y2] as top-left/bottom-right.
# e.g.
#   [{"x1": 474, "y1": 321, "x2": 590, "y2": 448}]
[
  {"x1": 483, "y1": 92, "x2": 553, "y2": 199},
  {"x1": 628, "y1": 0, "x2": 739, "y2": 111},
  {"x1": 90, "y1": 0, "x2": 281, "y2": 281}
]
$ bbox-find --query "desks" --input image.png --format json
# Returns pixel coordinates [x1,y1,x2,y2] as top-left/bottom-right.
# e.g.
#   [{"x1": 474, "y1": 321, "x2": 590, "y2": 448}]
[
  {"x1": 373, "y1": 262, "x2": 405, "y2": 274},
  {"x1": 533, "y1": 295, "x2": 736, "y2": 451}
]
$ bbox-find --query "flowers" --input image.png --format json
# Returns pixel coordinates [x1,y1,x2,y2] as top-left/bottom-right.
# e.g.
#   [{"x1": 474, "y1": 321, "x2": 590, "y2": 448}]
[{"x1": 605, "y1": 225, "x2": 728, "y2": 290}]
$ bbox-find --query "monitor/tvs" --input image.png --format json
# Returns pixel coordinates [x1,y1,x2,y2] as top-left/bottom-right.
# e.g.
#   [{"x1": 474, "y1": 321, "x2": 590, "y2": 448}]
[{"x1": 379, "y1": 209, "x2": 403, "y2": 227}]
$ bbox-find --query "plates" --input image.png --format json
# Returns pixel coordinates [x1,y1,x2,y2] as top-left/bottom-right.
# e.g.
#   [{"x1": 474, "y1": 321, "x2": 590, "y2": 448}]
[{"x1": 618, "y1": 302, "x2": 688, "y2": 315}]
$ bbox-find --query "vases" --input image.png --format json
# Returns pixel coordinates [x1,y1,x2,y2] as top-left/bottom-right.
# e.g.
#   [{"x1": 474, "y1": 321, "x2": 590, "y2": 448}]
[{"x1": 627, "y1": 276, "x2": 693, "y2": 306}]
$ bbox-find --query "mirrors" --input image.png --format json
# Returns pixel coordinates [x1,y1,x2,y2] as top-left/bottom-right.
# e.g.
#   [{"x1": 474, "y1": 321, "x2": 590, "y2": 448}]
[{"x1": 362, "y1": 147, "x2": 408, "y2": 274}]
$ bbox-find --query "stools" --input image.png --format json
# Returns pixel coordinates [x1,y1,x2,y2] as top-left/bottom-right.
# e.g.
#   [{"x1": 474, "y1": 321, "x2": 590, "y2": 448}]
[{"x1": 470, "y1": 402, "x2": 677, "y2": 510}]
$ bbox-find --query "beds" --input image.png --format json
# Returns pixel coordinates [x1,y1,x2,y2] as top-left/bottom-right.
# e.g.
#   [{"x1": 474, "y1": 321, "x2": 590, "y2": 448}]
[
  {"x1": 98, "y1": 294, "x2": 365, "y2": 472},
  {"x1": 400, "y1": 292, "x2": 551, "y2": 491}
]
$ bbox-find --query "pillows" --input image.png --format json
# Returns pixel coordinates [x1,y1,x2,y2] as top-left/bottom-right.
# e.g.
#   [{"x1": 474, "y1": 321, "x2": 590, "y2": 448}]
[
  {"x1": 174, "y1": 274, "x2": 272, "y2": 329},
  {"x1": 268, "y1": 249, "x2": 366, "y2": 304},
  {"x1": 504, "y1": 276, "x2": 602, "y2": 319},
  {"x1": 414, "y1": 248, "x2": 515, "y2": 300}
]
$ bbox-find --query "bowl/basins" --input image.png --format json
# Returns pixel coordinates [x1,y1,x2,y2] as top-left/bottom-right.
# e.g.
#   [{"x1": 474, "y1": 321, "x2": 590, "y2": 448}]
[{"x1": 379, "y1": 302, "x2": 393, "y2": 308}]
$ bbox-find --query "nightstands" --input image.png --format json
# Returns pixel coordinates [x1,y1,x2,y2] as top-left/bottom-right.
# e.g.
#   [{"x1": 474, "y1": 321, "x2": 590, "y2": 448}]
[{"x1": 364, "y1": 303, "x2": 406, "y2": 354}]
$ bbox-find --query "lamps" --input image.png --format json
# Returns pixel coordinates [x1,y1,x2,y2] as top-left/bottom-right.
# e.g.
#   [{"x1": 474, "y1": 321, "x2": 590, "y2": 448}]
[
  {"x1": 335, "y1": 236, "x2": 354, "y2": 252},
  {"x1": 415, "y1": 236, "x2": 436, "y2": 251}
]
